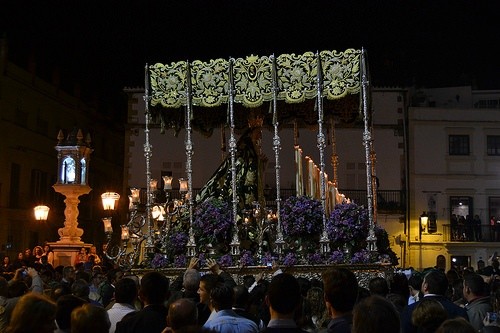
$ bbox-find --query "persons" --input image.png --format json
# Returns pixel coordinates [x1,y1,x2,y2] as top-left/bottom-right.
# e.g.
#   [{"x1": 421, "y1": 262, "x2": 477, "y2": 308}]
[
  {"x1": 195, "y1": 114, "x2": 270, "y2": 209},
  {"x1": 0, "y1": 246, "x2": 500, "y2": 333},
  {"x1": 451, "y1": 214, "x2": 500, "y2": 241}
]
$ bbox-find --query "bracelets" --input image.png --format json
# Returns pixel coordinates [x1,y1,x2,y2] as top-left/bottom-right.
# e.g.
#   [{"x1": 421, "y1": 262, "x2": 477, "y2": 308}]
[{"x1": 33, "y1": 274, "x2": 38, "y2": 278}]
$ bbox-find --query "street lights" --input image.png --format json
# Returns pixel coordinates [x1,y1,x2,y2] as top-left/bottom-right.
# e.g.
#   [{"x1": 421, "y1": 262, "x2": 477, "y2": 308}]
[{"x1": 45, "y1": 128, "x2": 94, "y2": 245}]
